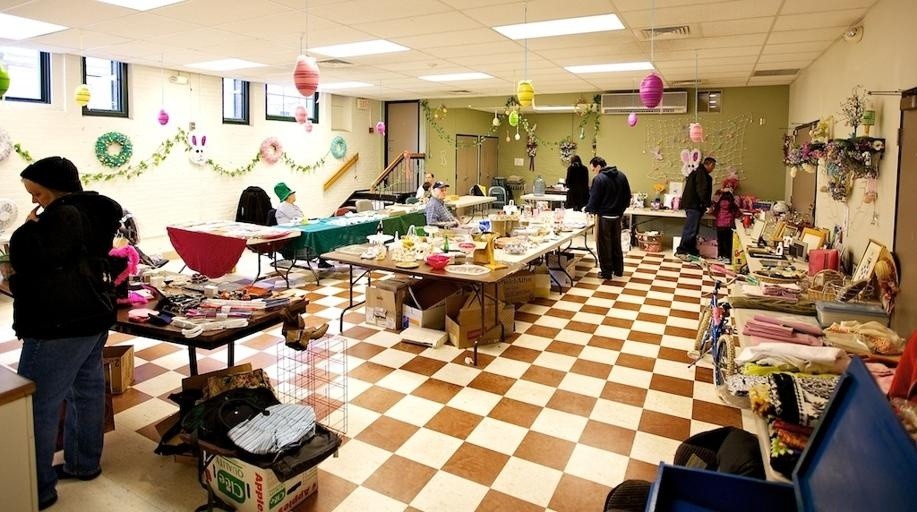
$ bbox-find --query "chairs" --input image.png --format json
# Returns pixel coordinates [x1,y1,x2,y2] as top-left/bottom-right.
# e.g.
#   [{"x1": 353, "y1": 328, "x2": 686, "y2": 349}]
[
  {"x1": 355, "y1": 199, "x2": 373, "y2": 212},
  {"x1": 400, "y1": 211, "x2": 426, "y2": 236},
  {"x1": 488, "y1": 186, "x2": 506, "y2": 210},
  {"x1": 335, "y1": 209, "x2": 349, "y2": 216}
]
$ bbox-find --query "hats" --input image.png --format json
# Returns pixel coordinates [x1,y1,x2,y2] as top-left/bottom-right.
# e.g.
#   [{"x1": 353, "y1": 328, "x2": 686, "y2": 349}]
[
  {"x1": 724, "y1": 184, "x2": 733, "y2": 193},
  {"x1": 20, "y1": 156, "x2": 83, "y2": 194},
  {"x1": 750, "y1": 373, "x2": 839, "y2": 428},
  {"x1": 432, "y1": 181, "x2": 449, "y2": 190},
  {"x1": 273, "y1": 182, "x2": 296, "y2": 203},
  {"x1": 874, "y1": 247, "x2": 899, "y2": 316}
]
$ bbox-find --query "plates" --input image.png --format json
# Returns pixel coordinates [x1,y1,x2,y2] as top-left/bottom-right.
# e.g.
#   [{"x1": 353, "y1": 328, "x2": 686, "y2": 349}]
[
  {"x1": 443, "y1": 264, "x2": 491, "y2": 275},
  {"x1": 440, "y1": 243, "x2": 460, "y2": 251},
  {"x1": 395, "y1": 261, "x2": 419, "y2": 269}
]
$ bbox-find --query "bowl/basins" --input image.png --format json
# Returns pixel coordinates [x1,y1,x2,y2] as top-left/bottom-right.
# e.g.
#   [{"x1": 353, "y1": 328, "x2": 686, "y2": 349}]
[
  {"x1": 426, "y1": 255, "x2": 450, "y2": 270},
  {"x1": 459, "y1": 242, "x2": 476, "y2": 253}
]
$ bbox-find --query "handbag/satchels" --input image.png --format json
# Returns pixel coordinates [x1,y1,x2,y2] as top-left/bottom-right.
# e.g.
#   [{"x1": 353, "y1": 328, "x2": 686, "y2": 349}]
[{"x1": 220, "y1": 397, "x2": 316, "y2": 455}]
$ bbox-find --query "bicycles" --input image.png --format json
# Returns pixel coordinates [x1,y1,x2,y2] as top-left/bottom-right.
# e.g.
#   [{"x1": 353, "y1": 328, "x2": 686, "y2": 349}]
[{"x1": 689, "y1": 261, "x2": 737, "y2": 391}]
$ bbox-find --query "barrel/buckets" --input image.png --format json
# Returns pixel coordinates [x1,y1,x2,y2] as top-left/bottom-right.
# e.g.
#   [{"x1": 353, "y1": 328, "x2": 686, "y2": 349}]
[{"x1": 532, "y1": 174, "x2": 546, "y2": 197}]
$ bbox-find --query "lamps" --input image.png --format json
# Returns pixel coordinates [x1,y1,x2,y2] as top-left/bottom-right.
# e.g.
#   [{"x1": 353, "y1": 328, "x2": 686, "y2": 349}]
[
  {"x1": 97, "y1": 0, "x2": 188, "y2": 12},
  {"x1": 183, "y1": 58, "x2": 268, "y2": 72},
  {"x1": 0, "y1": 13, "x2": 71, "y2": 41},
  {"x1": 491, "y1": 13, "x2": 626, "y2": 40},
  {"x1": 306, "y1": 39, "x2": 409, "y2": 58},
  {"x1": 563, "y1": 62, "x2": 656, "y2": 74},
  {"x1": 418, "y1": 71, "x2": 494, "y2": 83}
]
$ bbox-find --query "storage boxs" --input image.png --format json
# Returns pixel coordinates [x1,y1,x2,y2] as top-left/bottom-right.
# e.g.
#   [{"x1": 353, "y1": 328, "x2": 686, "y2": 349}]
[
  {"x1": 201, "y1": 449, "x2": 319, "y2": 512},
  {"x1": 402, "y1": 288, "x2": 470, "y2": 330},
  {"x1": 549, "y1": 252, "x2": 575, "y2": 282},
  {"x1": 366, "y1": 279, "x2": 412, "y2": 330},
  {"x1": 103, "y1": 345, "x2": 135, "y2": 394},
  {"x1": 444, "y1": 293, "x2": 514, "y2": 349}
]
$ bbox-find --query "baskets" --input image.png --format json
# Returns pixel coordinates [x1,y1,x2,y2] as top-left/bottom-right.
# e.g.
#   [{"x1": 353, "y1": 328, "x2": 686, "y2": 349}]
[
  {"x1": 807, "y1": 269, "x2": 844, "y2": 302},
  {"x1": 634, "y1": 228, "x2": 664, "y2": 252}
]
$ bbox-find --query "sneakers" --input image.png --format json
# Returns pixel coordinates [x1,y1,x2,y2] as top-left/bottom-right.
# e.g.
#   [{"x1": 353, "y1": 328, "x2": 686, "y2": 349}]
[
  {"x1": 718, "y1": 256, "x2": 722, "y2": 261},
  {"x1": 598, "y1": 271, "x2": 602, "y2": 278},
  {"x1": 39, "y1": 495, "x2": 57, "y2": 509},
  {"x1": 674, "y1": 252, "x2": 692, "y2": 262},
  {"x1": 53, "y1": 463, "x2": 70, "y2": 480},
  {"x1": 317, "y1": 261, "x2": 335, "y2": 269},
  {"x1": 698, "y1": 255, "x2": 705, "y2": 258},
  {"x1": 723, "y1": 256, "x2": 729, "y2": 263}
]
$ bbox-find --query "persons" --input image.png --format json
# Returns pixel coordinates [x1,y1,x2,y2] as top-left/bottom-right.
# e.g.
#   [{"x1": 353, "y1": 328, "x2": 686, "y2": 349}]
[
  {"x1": 674, "y1": 157, "x2": 716, "y2": 262},
  {"x1": 426, "y1": 182, "x2": 460, "y2": 225},
  {"x1": 415, "y1": 173, "x2": 435, "y2": 199},
  {"x1": 275, "y1": 182, "x2": 334, "y2": 269},
  {"x1": 711, "y1": 191, "x2": 742, "y2": 263},
  {"x1": 581, "y1": 157, "x2": 631, "y2": 279},
  {"x1": 422, "y1": 183, "x2": 431, "y2": 202},
  {"x1": 564, "y1": 155, "x2": 589, "y2": 211},
  {"x1": 0, "y1": 156, "x2": 123, "y2": 511}
]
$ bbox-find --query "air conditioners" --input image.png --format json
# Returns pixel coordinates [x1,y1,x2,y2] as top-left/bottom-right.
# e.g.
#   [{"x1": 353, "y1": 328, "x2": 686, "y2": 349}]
[{"x1": 601, "y1": 91, "x2": 687, "y2": 114}]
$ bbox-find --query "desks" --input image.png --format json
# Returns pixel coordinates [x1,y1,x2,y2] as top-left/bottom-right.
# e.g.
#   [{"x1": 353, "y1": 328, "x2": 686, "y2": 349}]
[
  {"x1": 167, "y1": 219, "x2": 301, "y2": 286},
  {"x1": 730, "y1": 286, "x2": 911, "y2": 479},
  {"x1": 0, "y1": 275, "x2": 310, "y2": 376},
  {"x1": 521, "y1": 193, "x2": 566, "y2": 201},
  {"x1": 385, "y1": 196, "x2": 496, "y2": 224},
  {"x1": 319, "y1": 211, "x2": 598, "y2": 367},
  {"x1": 0, "y1": 366, "x2": 38, "y2": 511},
  {"x1": 734, "y1": 218, "x2": 849, "y2": 286},
  {"x1": 272, "y1": 208, "x2": 427, "y2": 285},
  {"x1": 624, "y1": 207, "x2": 752, "y2": 251}
]
